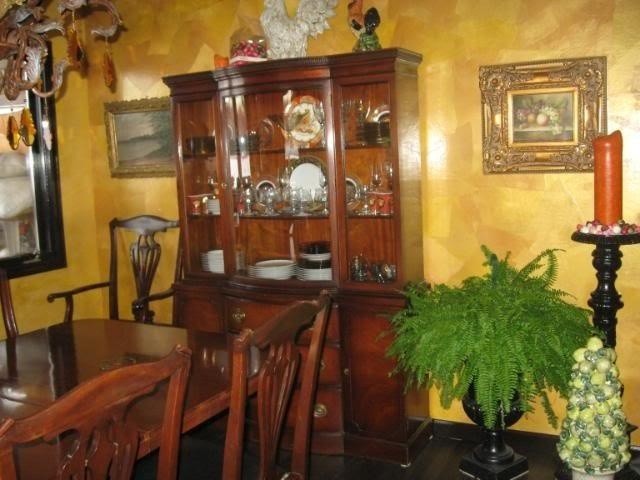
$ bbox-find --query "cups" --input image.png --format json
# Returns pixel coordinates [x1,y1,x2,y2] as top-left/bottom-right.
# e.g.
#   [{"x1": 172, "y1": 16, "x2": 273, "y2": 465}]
[
  {"x1": 240, "y1": 184, "x2": 327, "y2": 216},
  {"x1": 188, "y1": 192, "x2": 223, "y2": 215}
]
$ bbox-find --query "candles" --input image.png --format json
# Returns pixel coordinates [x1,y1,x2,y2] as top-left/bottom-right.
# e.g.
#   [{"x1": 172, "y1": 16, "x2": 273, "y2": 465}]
[{"x1": 592, "y1": 130, "x2": 623, "y2": 225}]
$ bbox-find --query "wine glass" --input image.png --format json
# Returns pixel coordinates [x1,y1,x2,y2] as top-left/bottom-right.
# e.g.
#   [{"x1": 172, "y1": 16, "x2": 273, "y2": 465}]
[
  {"x1": 319, "y1": 164, "x2": 331, "y2": 219},
  {"x1": 341, "y1": 95, "x2": 370, "y2": 147},
  {"x1": 359, "y1": 157, "x2": 394, "y2": 218},
  {"x1": 314, "y1": 102, "x2": 329, "y2": 149}
]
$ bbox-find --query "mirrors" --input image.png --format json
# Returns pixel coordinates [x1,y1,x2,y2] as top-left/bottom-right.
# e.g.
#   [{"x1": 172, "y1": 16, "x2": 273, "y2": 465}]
[{"x1": 0, "y1": 41, "x2": 67, "y2": 278}]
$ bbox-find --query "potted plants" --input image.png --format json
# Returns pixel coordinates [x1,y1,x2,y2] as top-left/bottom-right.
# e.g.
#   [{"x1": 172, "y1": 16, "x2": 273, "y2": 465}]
[{"x1": 375, "y1": 244, "x2": 607, "y2": 480}]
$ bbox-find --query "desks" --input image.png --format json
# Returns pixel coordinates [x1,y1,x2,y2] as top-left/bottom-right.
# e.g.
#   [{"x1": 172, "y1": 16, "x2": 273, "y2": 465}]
[{"x1": 0, "y1": 317, "x2": 302, "y2": 480}]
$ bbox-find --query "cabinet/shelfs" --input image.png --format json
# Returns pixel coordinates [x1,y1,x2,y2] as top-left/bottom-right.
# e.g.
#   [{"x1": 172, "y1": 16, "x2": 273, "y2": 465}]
[{"x1": 162, "y1": 47, "x2": 434, "y2": 468}]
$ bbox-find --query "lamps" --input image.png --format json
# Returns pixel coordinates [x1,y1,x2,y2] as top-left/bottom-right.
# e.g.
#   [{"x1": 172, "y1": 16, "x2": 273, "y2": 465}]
[{"x1": 1, "y1": 0, "x2": 123, "y2": 150}]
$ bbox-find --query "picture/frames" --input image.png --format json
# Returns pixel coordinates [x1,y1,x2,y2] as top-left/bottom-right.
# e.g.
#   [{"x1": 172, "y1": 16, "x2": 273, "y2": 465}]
[
  {"x1": 103, "y1": 96, "x2": 177, "y2": 180},
  {"x1": 477, "y1": 55, "x2": 608, "y2": 175}
]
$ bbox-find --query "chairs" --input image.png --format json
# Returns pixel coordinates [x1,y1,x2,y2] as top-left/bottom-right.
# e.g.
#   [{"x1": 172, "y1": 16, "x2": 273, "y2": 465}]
[
  {"x1": 0, "y1": 267, "x2": 18, "y2": 338},
  {"x1": 0, "y1": 342, "x2": 193, "y2": 479},
  {"x1": 46, "y1": 215, "x2": 182, "y2": 326},
  {"x1": 130, "y1": 288, "x2": 333, "y2": 480}
]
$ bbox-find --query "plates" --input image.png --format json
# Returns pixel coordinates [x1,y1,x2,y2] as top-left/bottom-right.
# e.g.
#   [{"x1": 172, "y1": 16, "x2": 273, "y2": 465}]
[
  {"x1": 278, "y1": 155, "x2": 326, "y2": 212},
  {"x1": 199, "y1": 242, "x2": 335, "y2": 284},
  {"x1": 343, "y1": 170, "x2": 363, "y2": 215},
  {"x1": 281, "y1": 95, "x2": 324, "y2": 149},
  {"x1": 250, "y1": 175, "x2": 282, "y2": 213}
]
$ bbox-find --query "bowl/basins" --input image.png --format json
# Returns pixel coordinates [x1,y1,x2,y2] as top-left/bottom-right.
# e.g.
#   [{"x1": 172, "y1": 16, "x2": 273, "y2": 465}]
[{"x1": 185, "y1": 137, "x2": 215, "y2": 155}]
